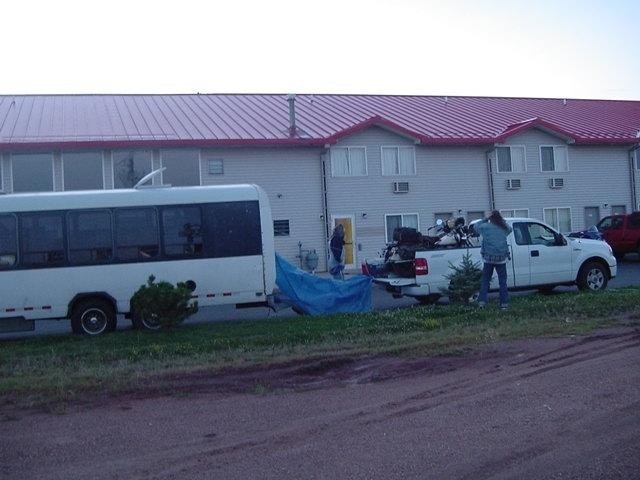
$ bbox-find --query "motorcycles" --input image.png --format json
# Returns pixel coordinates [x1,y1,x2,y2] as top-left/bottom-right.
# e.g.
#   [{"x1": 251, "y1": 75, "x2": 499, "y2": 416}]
[{"x1": 376, "y1": 209, "x2": 474, "y2": 278}]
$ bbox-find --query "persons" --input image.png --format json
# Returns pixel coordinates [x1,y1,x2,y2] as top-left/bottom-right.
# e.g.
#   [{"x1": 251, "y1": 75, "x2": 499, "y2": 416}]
[
  {"x1": 327, "y1": 223, "x2": 349, "y2": 280},
  {"x1": 472, "y1": 209, "x2": 512, "y2": 311}
]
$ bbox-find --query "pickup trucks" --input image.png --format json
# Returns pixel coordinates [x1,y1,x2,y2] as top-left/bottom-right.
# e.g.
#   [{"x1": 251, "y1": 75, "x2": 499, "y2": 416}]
[
  {"x1": 361, "y1": 218, "x2": 618, "y2": 304},
  {"x1": 568, "y1": 214, "x2": 640, "y2": 259}
]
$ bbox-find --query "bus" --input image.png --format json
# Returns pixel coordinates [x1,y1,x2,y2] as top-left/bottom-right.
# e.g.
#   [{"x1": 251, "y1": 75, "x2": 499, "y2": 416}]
[{"x1": 0, "y1": 166, "x2": 279, "y2": 337}]
